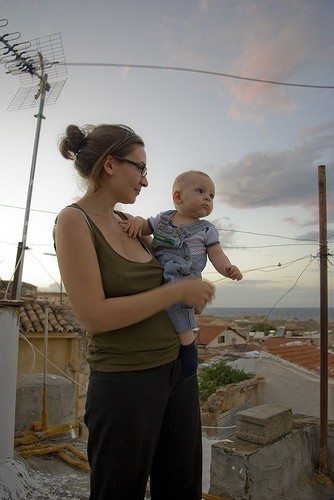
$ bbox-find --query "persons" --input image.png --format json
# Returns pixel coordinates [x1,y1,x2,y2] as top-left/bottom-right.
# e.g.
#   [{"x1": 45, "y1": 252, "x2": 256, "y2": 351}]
[
  {"x1": 116, "y1": 170, "x2": 243, "y2": 377},
  {"x1": 50, "y1": 121, "x2": 217, "y2": 500}
]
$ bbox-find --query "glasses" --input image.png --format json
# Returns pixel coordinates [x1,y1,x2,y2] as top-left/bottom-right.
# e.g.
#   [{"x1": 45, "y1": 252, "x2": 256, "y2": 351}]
[{"x1": 113, "y1": 155, "x2": 148, "y2": 179}]
